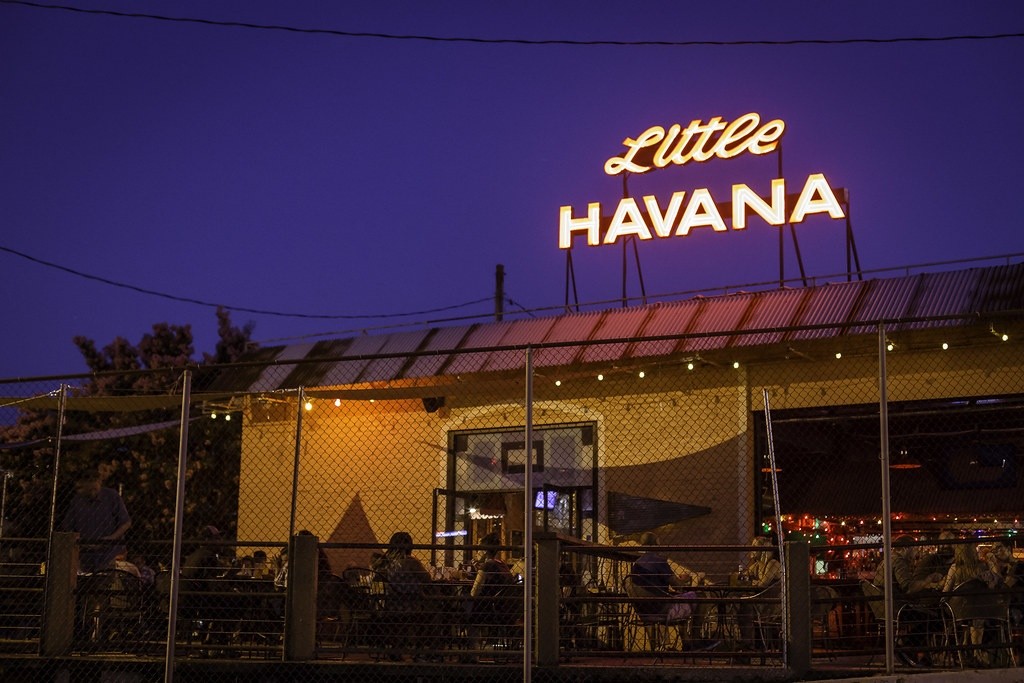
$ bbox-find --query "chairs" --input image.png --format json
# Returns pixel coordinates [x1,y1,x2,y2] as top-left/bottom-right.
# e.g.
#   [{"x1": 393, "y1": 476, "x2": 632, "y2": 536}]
[
  {"x1": 154, "y1": 570, "x2": 232, "y2": 658},
  {"x1": 316, "y1": 574, "x2": 345, "y2": 643},
  {"x1": 449, "y1": 578, "x2": 524, "y2": 665},
  {"x1": 1006, "y1": 602, "x2": 1024, "y2": 668},
  {"x1": 622, "y1": 574, "x2": 687, "y2": 666},
  {"x1": 341, "y1": 565, "x2": 405, "y2": 662},
  {"x1": 859, "y1": 578, "x2": 939, "y2": 668},
  {"x1": 821, "y1": 585, "x2": 841, "y2": 638},
  {"x1": 83, "y1": 569, "x2": 152, "y2": 660},
  {"x1": 940, "y1": 576, "x2": 1016, "y2": 670},
  {"x1": 729, "y1": 579, "x2": 781, "y2": 667},
  {"x1": 811, "y1": 584, "x2": 834, "y2": 662}
]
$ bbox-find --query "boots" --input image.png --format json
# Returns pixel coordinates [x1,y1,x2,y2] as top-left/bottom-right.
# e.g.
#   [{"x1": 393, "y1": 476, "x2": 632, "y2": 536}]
[
  {"x1": 957, "y1": 629, "x2": 969, "y2": 665},
  {"x1": 971, "y1": 626, "x2": 990, "y2": 665}
]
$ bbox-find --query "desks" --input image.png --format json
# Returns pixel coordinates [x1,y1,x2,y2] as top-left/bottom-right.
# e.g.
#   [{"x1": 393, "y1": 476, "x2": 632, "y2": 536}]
[
  {"x1": 427, "y1": 595, "x2": 475, "y2": 663},
  {"x1": 229, "y1": 575, "x2": 274, "y2": 660},
  {"x1": 681, "y1": 585, "x2": 768, "y2": 666}
]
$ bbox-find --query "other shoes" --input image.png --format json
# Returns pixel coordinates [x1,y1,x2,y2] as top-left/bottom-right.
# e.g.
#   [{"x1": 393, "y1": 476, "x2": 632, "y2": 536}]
[
  {"x1": 221, "y1": 649, "x2": 240, "y2": 658},
  {"x1": 896, "y1": 653, "x2": 916, "y2": 665}
]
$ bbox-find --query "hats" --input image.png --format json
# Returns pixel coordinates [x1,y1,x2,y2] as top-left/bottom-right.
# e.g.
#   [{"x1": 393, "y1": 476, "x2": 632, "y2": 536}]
[{"x1": 198, "y1": 526, "x2": 225, "y2": 540}]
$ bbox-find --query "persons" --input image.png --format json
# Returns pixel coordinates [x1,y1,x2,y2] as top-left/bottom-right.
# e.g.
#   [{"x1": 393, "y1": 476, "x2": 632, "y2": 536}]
[
  {"x1": 61, "y1": 469, "x2": 131, "y2": 649},
  {"x1": 42, "y1": 529, "x2": 1024, "y2": 668}
]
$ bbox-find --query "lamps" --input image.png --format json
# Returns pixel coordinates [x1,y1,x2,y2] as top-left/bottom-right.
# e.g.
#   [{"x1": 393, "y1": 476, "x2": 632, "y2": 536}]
[{"x1": 422, "y1": 397, "x2": 445, "y2": 413}]
